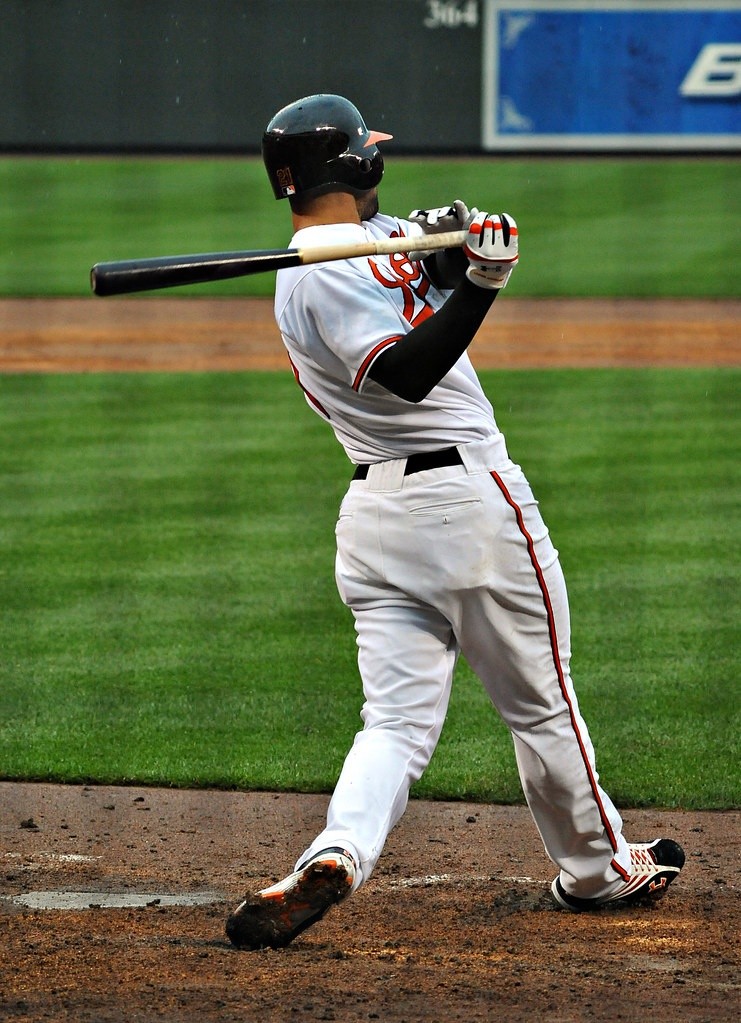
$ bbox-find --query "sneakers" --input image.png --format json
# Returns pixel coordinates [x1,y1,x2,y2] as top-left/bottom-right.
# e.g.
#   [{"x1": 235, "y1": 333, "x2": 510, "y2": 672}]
[
  {"x1": 224, "y1": 847, "x2": 357, "y2": 952},
  {"x1": 550, "y1": 837, "x2": 685, "y2": 912}
]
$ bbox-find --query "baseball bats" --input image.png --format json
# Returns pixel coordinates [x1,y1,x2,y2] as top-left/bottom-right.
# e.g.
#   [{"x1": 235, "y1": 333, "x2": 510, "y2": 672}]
[{"x1": 90, "y1": 228, "x2": 470, "y2": 298}]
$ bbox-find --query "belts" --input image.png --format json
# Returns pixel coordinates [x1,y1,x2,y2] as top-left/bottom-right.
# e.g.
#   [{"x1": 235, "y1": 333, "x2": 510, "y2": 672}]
[{"x1": 351, "y1": 446, "x2": 463, "y2": 480}]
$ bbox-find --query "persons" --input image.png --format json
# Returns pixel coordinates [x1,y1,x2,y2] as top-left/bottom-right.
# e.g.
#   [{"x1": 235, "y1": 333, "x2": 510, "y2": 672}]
[{"x1": 221, "y1": 92, "x2": 687, "y2": 950}]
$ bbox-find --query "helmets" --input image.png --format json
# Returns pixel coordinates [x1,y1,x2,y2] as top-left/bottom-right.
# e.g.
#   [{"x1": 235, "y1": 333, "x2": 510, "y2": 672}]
[{"x1": 261, "y1": 93, "x2": 393, "y2": 200}]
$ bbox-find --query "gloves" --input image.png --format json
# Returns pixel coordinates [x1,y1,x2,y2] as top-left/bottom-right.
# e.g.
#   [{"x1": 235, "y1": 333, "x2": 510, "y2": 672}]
[
  {"x1": 408, "y1": 200, "x2": 470, "y2": 291},
  {"x1": 461, "y1": 206, "x2": 520, "y2": 290}
]
